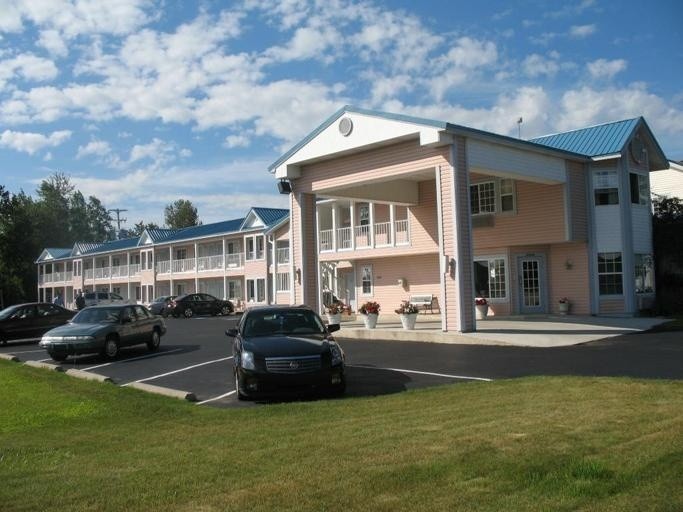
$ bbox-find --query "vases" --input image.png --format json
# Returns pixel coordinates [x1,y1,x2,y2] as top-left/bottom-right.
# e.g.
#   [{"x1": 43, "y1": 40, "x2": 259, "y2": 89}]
[
  {"x1": 326, "y1": 313, "x2": 341, "y2": 327},
  {"x1": 362, "y1": 314, "x2": 378, "y2": 328},
  {"x1": 399, "y1": 313, "x2": 417, "y2": 331},
  {"x1": 475, "y1": 305, "x2": 487, "y2": 319},
  {"x1": 559, "y1": 303, "x2": 570, "y2": 313}
]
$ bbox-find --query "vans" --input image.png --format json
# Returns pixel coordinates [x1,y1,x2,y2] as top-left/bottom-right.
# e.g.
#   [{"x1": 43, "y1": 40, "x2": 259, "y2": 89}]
[{"x1": 73, "y1": 292, "x2": 138, "y2": 311}]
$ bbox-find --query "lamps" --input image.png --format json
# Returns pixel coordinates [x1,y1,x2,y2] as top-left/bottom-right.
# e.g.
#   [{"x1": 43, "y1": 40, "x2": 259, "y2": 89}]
[{"x1": 277, "y1": 178, "x2": 290, "y2": 195}]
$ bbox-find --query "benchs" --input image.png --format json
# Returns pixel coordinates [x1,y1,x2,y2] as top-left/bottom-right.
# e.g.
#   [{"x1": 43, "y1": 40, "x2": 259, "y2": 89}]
[{"x1": 406, "y1": 294, "x2": 434, "y2": 315}]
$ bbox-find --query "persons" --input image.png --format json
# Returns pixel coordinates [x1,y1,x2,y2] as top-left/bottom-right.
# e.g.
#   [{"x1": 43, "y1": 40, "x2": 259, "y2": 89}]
[
  {"x1": 53, "y1": 293, "x2": 65, "y2": 307},
  {"x1": 76, "y1": 292, "x2": 85, "y2": 310}
]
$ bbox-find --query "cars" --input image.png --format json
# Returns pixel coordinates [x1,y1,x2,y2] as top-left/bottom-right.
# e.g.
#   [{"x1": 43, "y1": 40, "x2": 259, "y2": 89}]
[
  {"x1": 0, "y1": 302, "x2": 78, "y2": 345},
  {"x1": 224, "y1": 305, "x2": 348, "y2": 400},
  {"x1": 37, "y1": 303, "x2": 168, "y2": 362},
  {"x1": 166, "y1": 292, "x2": 233, "y2": 317},
  {"x1": 147, "y1": 295, "x2": 179, "y2": 318}
]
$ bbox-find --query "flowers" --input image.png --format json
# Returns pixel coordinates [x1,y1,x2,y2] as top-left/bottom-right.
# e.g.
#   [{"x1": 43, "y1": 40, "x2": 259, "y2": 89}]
[
  {"x1": 395, "y1": 300, "x2": 419, "y2": 315},
  {"x1": 358, "y1": 301, "x2": 381, "y2": 316},
  {"x1": 474, "y1": 298, "x2": 488, "y2": 305},
  {"x1": 324, "y1": 305, "x2": 341, "y2": 314},
  {"x1": 558, "y1": 296, "x2": 569, "y2": 304}
]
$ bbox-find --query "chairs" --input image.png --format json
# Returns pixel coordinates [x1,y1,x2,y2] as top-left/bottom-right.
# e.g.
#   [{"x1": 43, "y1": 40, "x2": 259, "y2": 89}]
[
  {"x1": 282, "y1": 315, "x2": 305, "y2": 334},
  {"x1": 248, "y1": 319, "x2": 276, "y2": 334},
  {"x1": 21, "y1": 309, "x2": 29, "y2": 318}
]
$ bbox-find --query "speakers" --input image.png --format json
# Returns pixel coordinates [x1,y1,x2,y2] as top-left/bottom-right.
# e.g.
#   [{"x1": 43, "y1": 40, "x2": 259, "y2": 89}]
[{"x1": 278, "y1": 182, "x2": 291, "y2": 194}]
